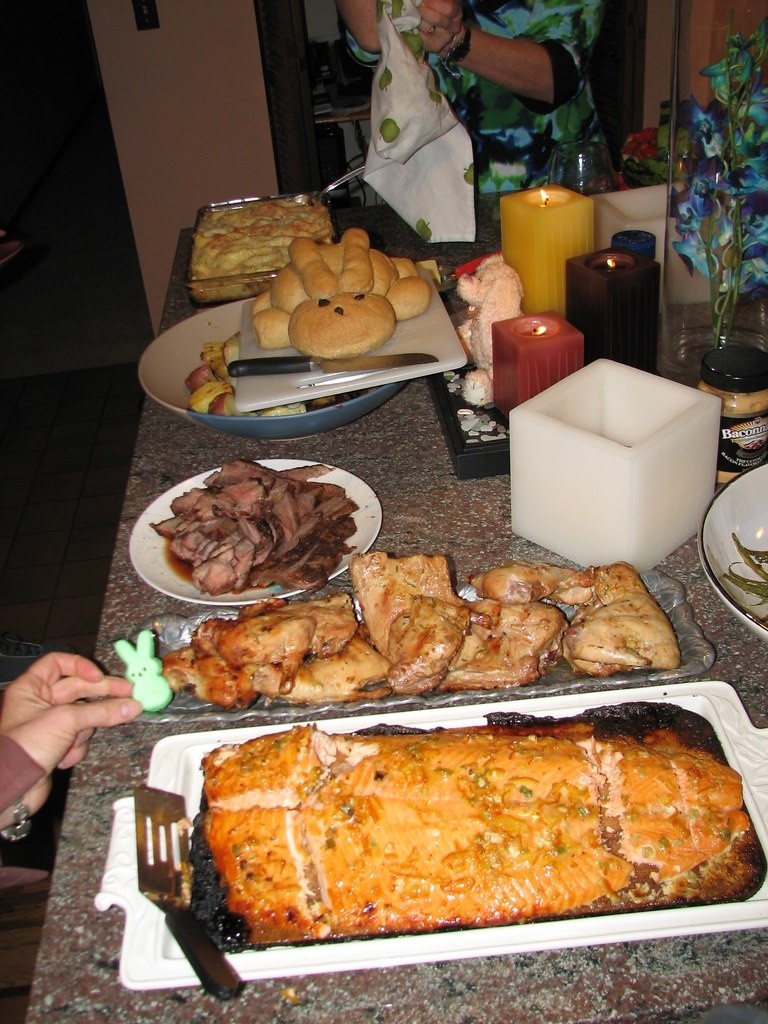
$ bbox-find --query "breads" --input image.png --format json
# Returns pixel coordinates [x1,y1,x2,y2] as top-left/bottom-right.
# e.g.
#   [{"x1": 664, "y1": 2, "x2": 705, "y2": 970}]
[{"x1": 248, "y1": 227, "x2": 429, "y2": 360}]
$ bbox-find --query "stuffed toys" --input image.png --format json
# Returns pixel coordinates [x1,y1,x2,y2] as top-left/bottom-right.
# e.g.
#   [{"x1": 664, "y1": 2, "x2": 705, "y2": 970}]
[{"x1": 453, "y1": 254, "x2": 524, "y2": 406}]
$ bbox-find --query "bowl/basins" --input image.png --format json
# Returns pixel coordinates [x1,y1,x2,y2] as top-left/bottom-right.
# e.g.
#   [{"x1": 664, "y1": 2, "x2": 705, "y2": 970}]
[
  {"x1": 697, "y1": 462, "x2": 768, "y2": 644},
  {"x1": 137, "y1": 295, "x2": 410, "y2": 439}
]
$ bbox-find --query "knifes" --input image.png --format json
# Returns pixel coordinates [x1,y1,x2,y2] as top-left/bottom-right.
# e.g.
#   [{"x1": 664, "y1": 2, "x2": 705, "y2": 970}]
[{"x1": 227, "y1": 352, "x2": 439, "y2": 376}]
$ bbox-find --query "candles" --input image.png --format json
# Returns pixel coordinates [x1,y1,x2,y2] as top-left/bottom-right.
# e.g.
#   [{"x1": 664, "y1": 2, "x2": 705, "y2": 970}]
[
  {"x1": 498, "y1": 184, "x2": 594, "y2": 318},
  {"x1": 490, "y1": 309, "x2": 583, "y2": 418},
  {"x1": 562, "y1": 245, "x2": 659, "y2": 376}
]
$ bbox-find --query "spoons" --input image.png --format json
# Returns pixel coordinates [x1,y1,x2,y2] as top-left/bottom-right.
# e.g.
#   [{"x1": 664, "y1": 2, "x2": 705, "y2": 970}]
[{"x1": 288, "y1": 166, "x2": 369, "y2": 207}]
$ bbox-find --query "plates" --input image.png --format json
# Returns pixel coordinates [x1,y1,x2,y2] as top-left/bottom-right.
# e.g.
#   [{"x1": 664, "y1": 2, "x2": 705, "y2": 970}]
[
  {"x1": 91, "y1": 680, "x2": 768, "y2": 991},
  {"x1": 126, "y1": 459, "x2": 383, "y2": 605},
  {"x1": 121, "y1": 570, "x2": 715, "y2": 724}
]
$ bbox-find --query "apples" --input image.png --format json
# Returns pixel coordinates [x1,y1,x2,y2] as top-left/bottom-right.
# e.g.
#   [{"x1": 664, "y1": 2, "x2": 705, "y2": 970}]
[{"x1": 190, "y1": 330, "x2": 249, "y2": 418}]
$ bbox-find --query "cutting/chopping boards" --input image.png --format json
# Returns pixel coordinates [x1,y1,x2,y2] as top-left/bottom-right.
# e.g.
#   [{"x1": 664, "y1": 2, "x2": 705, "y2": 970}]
[{"x1": 237, "y1": 268, "x2": 469, "y2": 414}]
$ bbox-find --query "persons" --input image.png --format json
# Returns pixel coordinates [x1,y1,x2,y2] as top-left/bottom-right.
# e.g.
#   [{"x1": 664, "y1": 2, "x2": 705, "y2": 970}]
[
  {"x1": 0, "y1": 651, "x2": 142, "y2": 888},
  {"x1": 335, "y1": 0, "x2": 613, "y2": 195}
]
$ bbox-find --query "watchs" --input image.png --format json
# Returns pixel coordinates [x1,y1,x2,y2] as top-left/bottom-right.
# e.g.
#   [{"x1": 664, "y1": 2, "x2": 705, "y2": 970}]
[{"x1": 448, "y1": 24, "x2": 471, "y2": 61}]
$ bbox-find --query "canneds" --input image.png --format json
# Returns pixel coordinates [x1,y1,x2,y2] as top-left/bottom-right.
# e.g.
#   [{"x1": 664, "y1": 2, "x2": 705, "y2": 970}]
[{"x1": 695, "y1": 345, "x2": 768, "y2": 484}]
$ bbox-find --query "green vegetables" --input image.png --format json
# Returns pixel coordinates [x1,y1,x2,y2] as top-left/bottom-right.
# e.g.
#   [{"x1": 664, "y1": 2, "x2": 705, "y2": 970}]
[{"x1": 721, "y1": 531, "x2": 768, "y2": 625}]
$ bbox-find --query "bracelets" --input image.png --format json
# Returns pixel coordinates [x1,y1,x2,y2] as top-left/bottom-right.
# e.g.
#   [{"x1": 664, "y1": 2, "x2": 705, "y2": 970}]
[
  {"x1": 1, "y1": 800, "x2": 30, "y2": 842},
  {"x1": 442, "y1": 36, "x2": 463, "y2": 79}
]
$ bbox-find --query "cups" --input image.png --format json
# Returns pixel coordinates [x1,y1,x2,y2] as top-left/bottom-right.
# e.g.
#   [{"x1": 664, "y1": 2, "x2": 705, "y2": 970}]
[{"x1": 548, "y1": 140, "x2": 613, "y2": 198}]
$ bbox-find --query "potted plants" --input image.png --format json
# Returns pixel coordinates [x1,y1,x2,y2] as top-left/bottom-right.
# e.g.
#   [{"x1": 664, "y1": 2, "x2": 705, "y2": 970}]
[{"x1": 646, "y1": 6, "x2": 767, "y2": 375}]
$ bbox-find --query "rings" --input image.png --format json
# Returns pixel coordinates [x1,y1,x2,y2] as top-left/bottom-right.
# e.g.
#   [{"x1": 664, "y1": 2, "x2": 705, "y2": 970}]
[{"x1": 429, "y1": 26, "x2": 435, "y2": 33}]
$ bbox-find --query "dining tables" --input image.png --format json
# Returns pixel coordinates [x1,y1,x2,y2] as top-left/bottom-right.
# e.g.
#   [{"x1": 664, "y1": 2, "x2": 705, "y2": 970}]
[{"x1": 20, "y1": 214, "x2": 765, "y2": 1024}]
